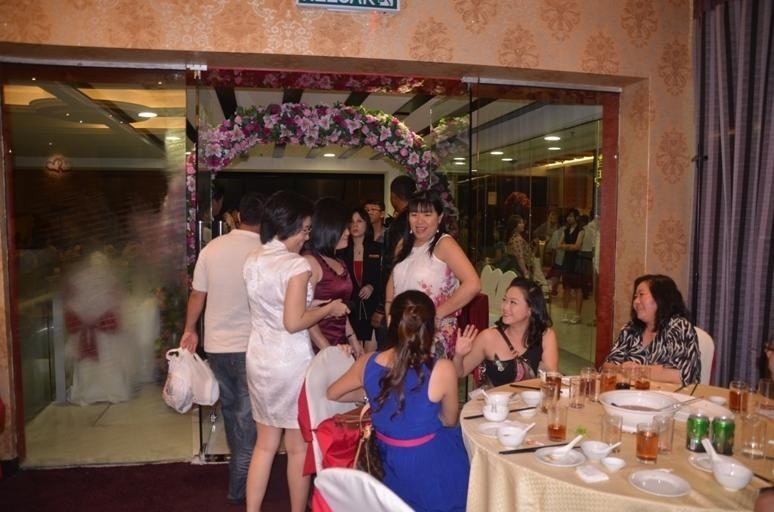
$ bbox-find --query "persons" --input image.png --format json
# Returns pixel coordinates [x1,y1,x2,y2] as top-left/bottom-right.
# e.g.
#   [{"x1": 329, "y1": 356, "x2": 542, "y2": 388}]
[
  {"x1": 577, "y1": 215, "x2": 594, "y2": 300},
  {"x1": 493, "y1": 243, "x2": 525, "y2": 280},
  {"x1": 302, "y1": 195, "x2": 365, "y2": 360},
  {"x1": 327, "y1": 290, "x2": 469, "y2": 512},
  {"x1": 198, "y1": 184, "x2": 226, "y2": 235},
  {"x1": 506, "y1": 215, "x2": 534, "y2": 279},
  {"x1": 380, "y1": 174, "x2": 416, "y2": 276},
  {"x1": 454, "y1": 277, "x2": 558, "y2": 386},
  {"x1": 558, "y1": 208, "x2": 584, "y2": 324},
  {"x1": 362, "y1": 198, "x2": 387, "y2": 245},
  {"x1": 338, "y1": 207, "x2": 384, "y2": 353},
  {"x1": 383, "y1": 190, "x2": 482, "y2": 361},
  {"x1": 242, "y1": 193, "x2": 350, "y2": 512},
  {"x1": 602, "y1": 274, "x2": 701, "y2": 388},
  {"x1": 180, "y1": 194, "x2": 263, "y2": 504},
  {"x1": 548, "y1": 217, "x2": 569, "y2": 299}
]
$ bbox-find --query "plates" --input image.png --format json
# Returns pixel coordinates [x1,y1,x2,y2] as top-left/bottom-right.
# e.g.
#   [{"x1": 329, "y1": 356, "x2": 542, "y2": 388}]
[
  {"x1": 475, "y1": 420, "x2": 526, "y2": 439},
  {"x1": 534, "y1": 446, "x2": 585, "y2": 468},
  {"x1": 628, "y1": 469, "x2": 690, "y2": 498},
  {"x1": 651, "y1": 391, "x2": 735, "y2": 424},
  {"x1": 687, "y1": 451, "x2": 739, "y2": 473}
]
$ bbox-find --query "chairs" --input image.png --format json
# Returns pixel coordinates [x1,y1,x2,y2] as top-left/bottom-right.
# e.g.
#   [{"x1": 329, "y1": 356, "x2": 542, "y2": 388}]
[
  {"x1": 310, "y1": 467, "x2": 416, "y2": 512},
  {"x1": 297, "y1": 345, "x2": 356, "y2": 477},
  {"x1": 693, "y1": 327, "x2": 717, "y2": 385},
  {"x1": 480, "y1": 264, "x2": 517, "y2": 315}
]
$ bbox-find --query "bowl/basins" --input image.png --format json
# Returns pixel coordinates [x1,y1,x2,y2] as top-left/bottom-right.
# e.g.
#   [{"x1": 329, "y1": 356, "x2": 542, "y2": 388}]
[
  {"x1": 519, "y1": 409, "x2": 536, "y2": 417},
  {"x1": 710, "y1": 395, "x2": 727, "y2": 405},
  {"x1": 496, "y1": 427, "x2": 525, "y2": 449},
  {"x1": 485, "y1": 392, "x2": 508, "y2": 406},
  {"x1": 581, "y1": 441, "x2": 609, "y2": 464},
  {"x1": 521, "y1": 390, "x2": 544, "y2": 406},
  {"x1": 712, "y1": 461, "x2": 753, "y2": 491},
  {"x1": 600, "y1": 457, "x2": 626, "y2": 472},
  {"x1": 482, "y1": 405, "x2": 508, "y2": 422},
  {"x1": 597, "y1": 388, "x2": 681, "y2": 435}
]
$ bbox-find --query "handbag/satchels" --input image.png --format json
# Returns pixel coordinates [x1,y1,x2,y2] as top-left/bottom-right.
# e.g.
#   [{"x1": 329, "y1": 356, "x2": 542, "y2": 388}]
[{"x1": 336, "y1": 402, "x2": 386, "y2": 482}]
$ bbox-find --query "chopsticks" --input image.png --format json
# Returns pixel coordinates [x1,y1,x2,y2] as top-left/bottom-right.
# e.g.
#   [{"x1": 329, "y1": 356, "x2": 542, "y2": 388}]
[
  {"x1": 463, "y1": 406, "x2": 537, "y2": 419},
  {"x1": 673, "y1": 382, "x2": 697, "y2": 396},
  {"x1": 509, "y1": 384, "x2": 562, "y2": 394},
  {"x1": 498, "y1": 442, "x2": 581, "y2": 455}
]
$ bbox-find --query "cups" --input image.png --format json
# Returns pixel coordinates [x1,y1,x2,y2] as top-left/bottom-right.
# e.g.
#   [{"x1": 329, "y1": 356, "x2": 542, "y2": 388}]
[
  {"x1": 540, "y1": 382, "x2": 557, "y2": 416},
  {"x1": 652, "y1": 415, "x2": 675, "y2": 455},
  {"x1": 740, "y1": 385, "x2": 760, "y2": 418},
  {"x1": 758, "y1": 379, "x2": 774, "y2": 410},
  {"x1": 634, "y1": 366, "x2": 652, "y2": 390},
  {"x1": 601, "y1": 367, "x2": 616, "y2": 391},
  {"x1": 580, "y1": 368, "x2": 593, "y2": 398},
  {"x1": 601, "y1": 414, "x2": 623, "y2": 455},
  {"x1": 728, "y1": 380, "x2": 750, "y2": 416},
  {"x1": 738, "y1": 415, "x2": 767, "y2": 461},
  {"x1": 636, "y1": 423, "x2": 659, "y2": 465},
  {"x1": 569, "y1": 378, "x2": 587, "y2": 411},
  {"x1": 545, "y1": 369, "x2": 562, "y2": 401},
  {"x1": 616, "y1": 366, "x2": 631, "y2": 389},
  {"x1": 547, "y1": 404, "x2": 567, "y2": 442},
  {"x1": 589, "y1": 372, "x2": 601, "y2": 401}
]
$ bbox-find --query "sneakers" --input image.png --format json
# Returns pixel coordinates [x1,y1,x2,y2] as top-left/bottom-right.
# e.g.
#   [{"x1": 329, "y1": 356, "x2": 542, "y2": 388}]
[
  {"x1": 570, "y1": 315, "x2": 582, "y2": 324},
  {"x1": 560, "y1": 313, "x2": 568, "y2": 322},
  {"x1": 585, "y1": 318, "x2": 597, "y2": 326}
]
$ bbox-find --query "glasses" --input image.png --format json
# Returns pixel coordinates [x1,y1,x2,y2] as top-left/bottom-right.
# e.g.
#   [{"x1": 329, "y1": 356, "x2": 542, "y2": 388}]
[{"x1": 302, "y1": 227, "x2": 312, "y2": 233}]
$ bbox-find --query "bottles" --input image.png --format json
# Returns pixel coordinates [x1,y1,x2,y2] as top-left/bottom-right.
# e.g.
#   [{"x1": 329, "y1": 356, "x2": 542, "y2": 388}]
[
  {"x1": 686, "y1": 412, "x2": 710, "y2": 454},
  {"x1": 711, "y1": 415, "x2": 735, "y2": 456}
]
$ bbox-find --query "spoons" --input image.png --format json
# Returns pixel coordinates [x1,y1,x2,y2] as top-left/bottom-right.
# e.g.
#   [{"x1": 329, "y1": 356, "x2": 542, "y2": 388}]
[{"x1": 551, "y1": 434, "x2": 581, "y2": 461}]
